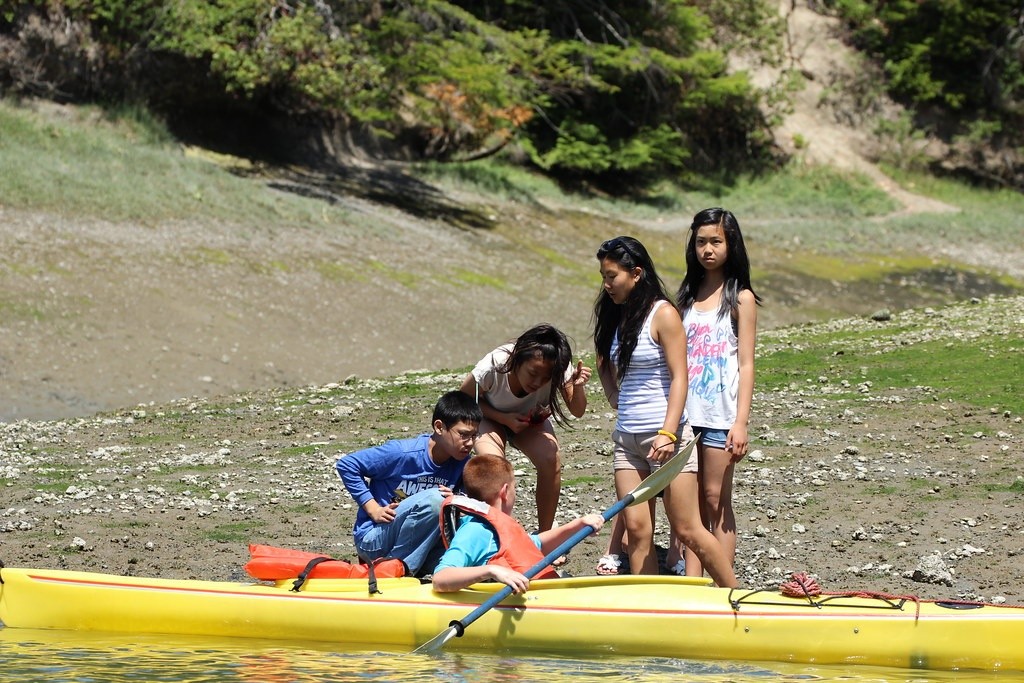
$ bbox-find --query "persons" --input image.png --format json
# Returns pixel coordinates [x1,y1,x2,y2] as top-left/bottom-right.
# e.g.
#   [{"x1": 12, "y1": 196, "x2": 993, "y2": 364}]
[
  {"x1": 588, "y1": 233, "x2": 742, "y2": 594},
  {"x1": 669, "y1": 207, "x2": 767, "y2": 588},
  {"x1": 335, "y1": 391, "x2": 487, "y2": 577},
  {"x1": 457, "y1": 322, "x2": 595, "y2": 563},
  {"x1": 596, "y1": 318, "x2": 697, "y2": 578},
  {"x1": 429, "y1": 450, "x2": 604, "y2": 600}
]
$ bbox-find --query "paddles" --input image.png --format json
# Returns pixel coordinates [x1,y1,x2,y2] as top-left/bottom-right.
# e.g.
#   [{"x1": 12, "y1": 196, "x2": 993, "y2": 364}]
[{"x1": 409, "y1": 433, "x2": 700, "y2": 654}]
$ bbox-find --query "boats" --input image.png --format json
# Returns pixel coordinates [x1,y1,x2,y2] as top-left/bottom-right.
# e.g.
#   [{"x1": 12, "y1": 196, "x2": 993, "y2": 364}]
[{"x1": 1, "y1": 544, "x2": 1023, "y2": 678}]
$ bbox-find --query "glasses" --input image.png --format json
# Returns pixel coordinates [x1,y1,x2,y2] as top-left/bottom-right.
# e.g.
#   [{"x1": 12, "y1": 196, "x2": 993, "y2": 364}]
[
  {"x1": 443, "y1": 421, "x2": 482, "y2": 441},
  {"x1": 600, "y1": 238, "x2": 638, "y2": 265}
]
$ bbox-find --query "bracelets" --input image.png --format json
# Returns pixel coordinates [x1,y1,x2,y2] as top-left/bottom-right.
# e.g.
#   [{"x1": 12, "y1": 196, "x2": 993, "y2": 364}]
[{"x1": 656, "y1": 428, "x2": 678, "y2": 442}]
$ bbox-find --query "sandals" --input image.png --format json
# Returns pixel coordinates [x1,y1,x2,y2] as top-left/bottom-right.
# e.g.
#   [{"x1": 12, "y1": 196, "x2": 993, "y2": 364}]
[
  {"x1": 595, "y1": 553, "x2": 624, "y2": 575},
  {"x1": 665, "y1": 560, "x2": 685, "y2": 576}
]
